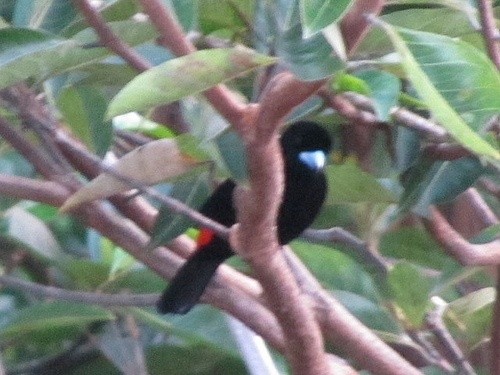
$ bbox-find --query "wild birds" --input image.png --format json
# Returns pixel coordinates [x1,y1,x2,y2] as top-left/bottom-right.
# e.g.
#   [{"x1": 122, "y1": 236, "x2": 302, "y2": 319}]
[{"x1": 154, "y1": 120, "x2": 333, "y2": 317}]
